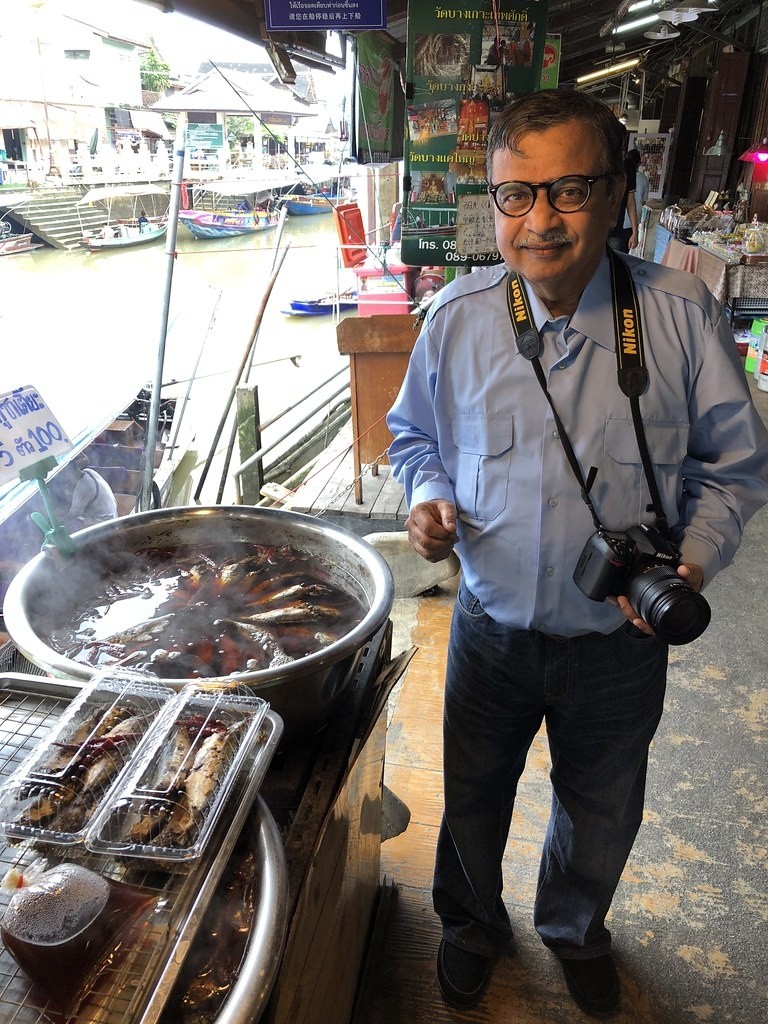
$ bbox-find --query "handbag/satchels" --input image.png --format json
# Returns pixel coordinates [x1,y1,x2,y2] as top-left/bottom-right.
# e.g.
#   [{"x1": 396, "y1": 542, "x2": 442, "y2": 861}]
[{"x1": 64, "y1": 509, "x2": 84, "y2": 534}]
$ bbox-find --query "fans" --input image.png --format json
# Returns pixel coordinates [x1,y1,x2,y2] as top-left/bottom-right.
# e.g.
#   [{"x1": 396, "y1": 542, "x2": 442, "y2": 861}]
[{"x1": 412, "y1": 274, "x2": 446, "y2": 312}]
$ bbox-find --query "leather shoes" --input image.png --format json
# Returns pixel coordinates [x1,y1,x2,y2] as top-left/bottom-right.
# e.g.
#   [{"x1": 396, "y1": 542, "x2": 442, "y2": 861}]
[
  {"x1": 435, "y1": 937, "x2": 497, "y2": 1006},
  {"x1": 561, "y1": 956, "x2": 623, "y2": 1020}
]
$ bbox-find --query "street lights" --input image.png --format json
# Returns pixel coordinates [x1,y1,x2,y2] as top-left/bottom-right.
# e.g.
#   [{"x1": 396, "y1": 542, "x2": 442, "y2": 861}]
[{"x1": 29, "y1": 0, "x2": 66, "y2": 183}]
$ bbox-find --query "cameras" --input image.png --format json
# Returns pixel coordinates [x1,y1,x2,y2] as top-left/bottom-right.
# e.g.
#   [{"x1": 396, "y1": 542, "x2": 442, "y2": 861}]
[{"x1": 573, "y1": 523, "x2": 712, "y2": 645}]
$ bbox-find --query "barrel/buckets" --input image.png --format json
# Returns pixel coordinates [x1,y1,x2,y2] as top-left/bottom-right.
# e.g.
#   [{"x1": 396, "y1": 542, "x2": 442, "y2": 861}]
[{"x1": 744, "y1": 317, "x2": 768, "y2": 392}]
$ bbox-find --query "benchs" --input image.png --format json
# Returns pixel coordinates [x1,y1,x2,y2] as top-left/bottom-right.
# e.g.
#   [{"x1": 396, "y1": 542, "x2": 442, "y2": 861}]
[{"x1": 50, "y1": 443, "x2": 164, "y2": 516}]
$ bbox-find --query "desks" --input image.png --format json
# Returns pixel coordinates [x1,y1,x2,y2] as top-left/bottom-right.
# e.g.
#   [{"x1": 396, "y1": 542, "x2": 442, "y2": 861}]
[
  {"x1": 1, "y1": 159, "x2": 29, "y2": 184},
  {"x1": 102, "y1": 420, "x2": 134, "y2": 446},
  {"x1": 628, "y1": 205, "x2": 768, "y2": 332},
  {"x1": 337, "y1": 313, "x2": 423, "y2": 505}
]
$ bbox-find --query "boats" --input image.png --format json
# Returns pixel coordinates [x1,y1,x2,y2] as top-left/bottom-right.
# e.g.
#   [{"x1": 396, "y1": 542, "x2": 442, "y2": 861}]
[
  {"x1": 0, "y1": 383, "x2": 191, "y2": 674},
  {"x1": 75, "y1": 184, "x2": 171, "y2": 251},
  {"x1": 280, "y1": 289, "x2": 358, "y2": 316},
  {"x1": 178, "y1": 179, "x2": 300, "y2": 240},
  {"x1": 0, "y1": 219, "x2": 44, "y2": 257},
  {"x1": 280, "y1": 164, "x2": 365, "y2": 215}
]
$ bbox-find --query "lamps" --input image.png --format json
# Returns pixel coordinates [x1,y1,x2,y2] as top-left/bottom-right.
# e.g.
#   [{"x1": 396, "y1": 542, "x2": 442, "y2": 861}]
[
  {"x1": 706, "y1": 17, "x2": 734, "y2": 157},
  {"x1": 737, "y1": 136, "x2": 768, "y2": 164}
]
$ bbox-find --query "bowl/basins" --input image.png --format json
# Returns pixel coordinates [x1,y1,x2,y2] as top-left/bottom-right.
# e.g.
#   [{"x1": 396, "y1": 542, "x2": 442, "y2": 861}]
[
  {"x1": 3, "y1": 506, "x2": 396, "y2": 709},
  {"x1": 213, "y1": 789, "x2": 291, "y2": 1022}
]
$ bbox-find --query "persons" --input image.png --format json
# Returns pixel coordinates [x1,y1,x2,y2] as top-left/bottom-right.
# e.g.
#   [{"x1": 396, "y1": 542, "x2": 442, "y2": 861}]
[
  {"x1": 487, "y1": 30, "x2": 531, "y2": 67},
  {"x1": 57, "y1": 452, "x2": 119, "y2": 528},
  {"x1": 475, "y1": 85, "x2": 500, "y2": 101},
  {"x1": 607, "y1": 122, "x2": 649, "y2": 254},
  {"x1": 99, "y1": 222, "x2": 115, "y2": 238},
  {"x1": 386, "y1": 88, "x2": 768, "y2": 1019},
  {"x1": 139, "y1": 211, "x2": 148, "y2": 233}
]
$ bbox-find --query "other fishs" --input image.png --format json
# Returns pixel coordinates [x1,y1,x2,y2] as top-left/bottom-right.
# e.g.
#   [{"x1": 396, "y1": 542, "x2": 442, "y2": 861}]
[
  {"x1": 42, "y1": 544, "x2": 366, "y2": 679},
  {"x1": 6, "y1": 706, "x2": 255, "y2": 870}
]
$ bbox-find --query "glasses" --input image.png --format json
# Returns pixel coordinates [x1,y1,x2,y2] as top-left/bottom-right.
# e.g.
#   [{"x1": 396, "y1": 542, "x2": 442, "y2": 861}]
[{"x1": 485, "y1": 170, "x2": 618, "y2": 217}]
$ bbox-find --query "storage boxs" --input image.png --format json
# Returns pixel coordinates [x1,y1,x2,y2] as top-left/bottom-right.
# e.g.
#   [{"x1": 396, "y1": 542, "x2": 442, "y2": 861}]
[
  {"x1": 334, "y1": 202, "x2": 446, "y2": 317},
  {"x1": 726, "y1": 297, "x2": 768, "y2": 315}
]
